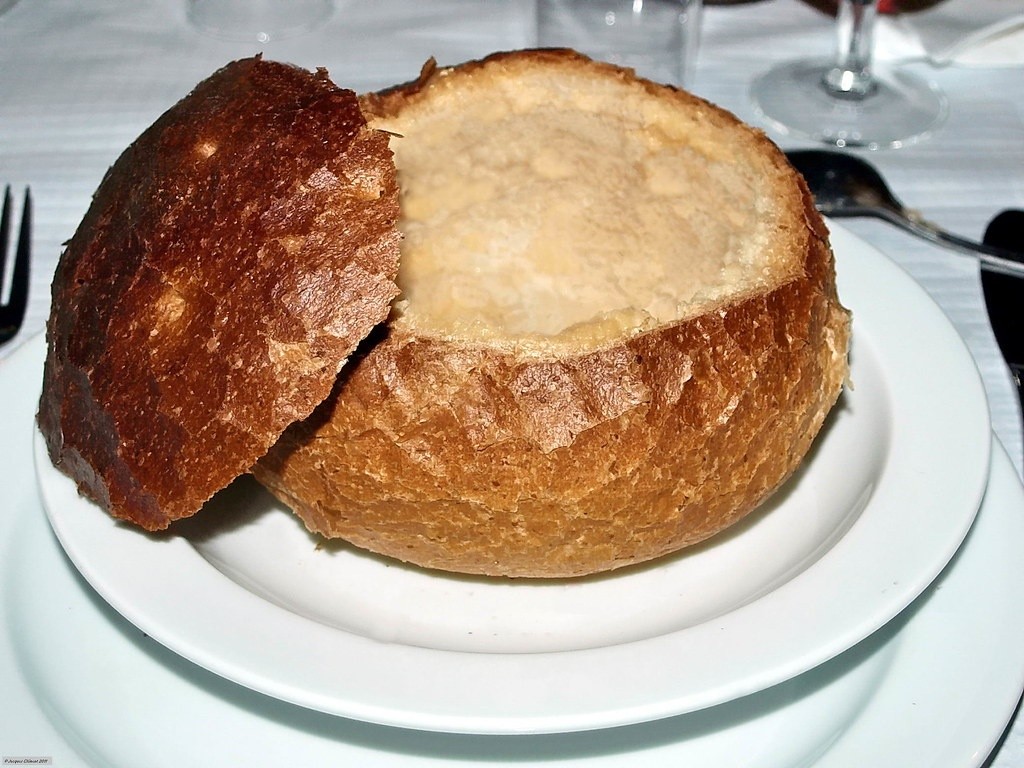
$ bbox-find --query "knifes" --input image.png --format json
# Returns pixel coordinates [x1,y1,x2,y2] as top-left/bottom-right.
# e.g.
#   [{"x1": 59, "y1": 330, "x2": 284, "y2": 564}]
[{"x1": 980, "y1": 207, "x2": 1024, "y2": 430}]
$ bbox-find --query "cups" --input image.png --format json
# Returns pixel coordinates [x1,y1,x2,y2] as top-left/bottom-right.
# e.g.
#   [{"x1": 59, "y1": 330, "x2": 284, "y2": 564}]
[{"x1": 536, "y1": 0, "x2": 702, "y2": 94}]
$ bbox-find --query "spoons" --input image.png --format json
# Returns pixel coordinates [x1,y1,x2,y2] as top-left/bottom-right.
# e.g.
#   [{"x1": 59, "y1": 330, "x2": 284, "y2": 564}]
[{"x1": 780, "y1": 149, "x2": 1024, "y2": 278}]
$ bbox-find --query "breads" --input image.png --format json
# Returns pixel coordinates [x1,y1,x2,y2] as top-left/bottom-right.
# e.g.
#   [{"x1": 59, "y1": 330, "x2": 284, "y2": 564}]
[{"x1": 35, "y1": 43, "x2": 853, "y2": 582}]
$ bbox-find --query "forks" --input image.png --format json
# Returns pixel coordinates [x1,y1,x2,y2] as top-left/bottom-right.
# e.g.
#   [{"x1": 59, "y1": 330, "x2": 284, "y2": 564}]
[{"x1": 0, "y1": 184, "x2": 31, "y2": 348}]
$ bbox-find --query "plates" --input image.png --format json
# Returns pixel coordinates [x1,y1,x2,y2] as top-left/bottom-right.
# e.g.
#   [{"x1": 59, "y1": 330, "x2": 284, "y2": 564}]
[
  {"x1": 35, "y1": 214, "x2": 992, "y2": 735},
  {"x1": 0, "y1": 311, "x2": 1024, "y2": 768}
]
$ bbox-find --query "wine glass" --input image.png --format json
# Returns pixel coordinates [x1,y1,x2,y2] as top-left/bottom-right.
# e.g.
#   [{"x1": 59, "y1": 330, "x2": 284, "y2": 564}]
[{"x1": 752, "y1": 0, "x2": 949, "y2": 147}]
